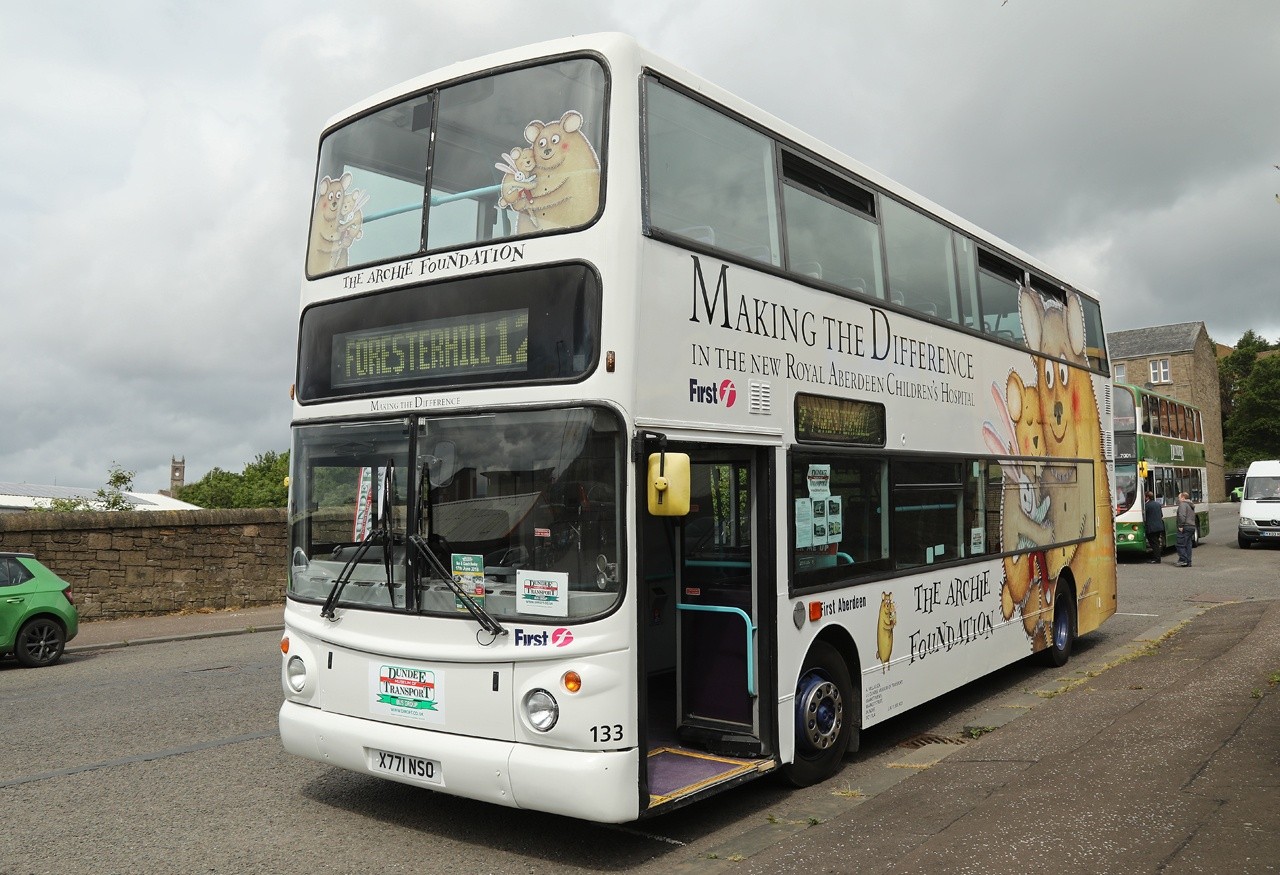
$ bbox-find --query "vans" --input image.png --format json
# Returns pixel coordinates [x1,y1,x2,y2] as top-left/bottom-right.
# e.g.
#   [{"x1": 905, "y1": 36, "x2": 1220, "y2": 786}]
[{"x1": 1238, "y1": 459, "x2": 1280, "y2": 549}]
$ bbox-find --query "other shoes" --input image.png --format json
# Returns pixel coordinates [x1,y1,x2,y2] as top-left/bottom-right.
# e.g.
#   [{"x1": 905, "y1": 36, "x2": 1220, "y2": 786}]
[
  {"x1": 1172, "y1": 560, "x2": 1191, "y2": 567},
  {"x1": 1150, "y1": 560, "x2": 1161, "y2": 564}
]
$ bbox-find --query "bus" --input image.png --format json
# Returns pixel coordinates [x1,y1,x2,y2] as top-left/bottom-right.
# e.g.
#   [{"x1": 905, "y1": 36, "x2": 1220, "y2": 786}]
[
  {"x1": 1112, "y1": 382, "x2": 1211, "y2": 555},
  {"x1": 280, "y1": 31, "x2": 1120, "y2": 825}
]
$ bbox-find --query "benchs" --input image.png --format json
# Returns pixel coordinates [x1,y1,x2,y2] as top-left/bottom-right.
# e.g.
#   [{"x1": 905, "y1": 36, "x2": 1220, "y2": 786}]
[{"x1": 674, "y1": 226, "x2": 1015, "y2": 345}]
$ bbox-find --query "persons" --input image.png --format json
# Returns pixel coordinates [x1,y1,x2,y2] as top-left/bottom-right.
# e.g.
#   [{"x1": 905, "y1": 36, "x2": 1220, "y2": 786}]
[
  {"x1": 1145, "y1": 492, "x2": 1163, "y2": 564},
  {"x1": 1173, "y1": 492, "x2": 1195, "y2": 567}
]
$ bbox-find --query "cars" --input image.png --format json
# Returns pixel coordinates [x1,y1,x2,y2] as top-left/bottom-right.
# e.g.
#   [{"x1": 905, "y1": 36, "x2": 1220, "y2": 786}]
[
  {"x1": 1226, "y1": 487, "x2": 1243, "y2": 502},
  {"x1": 0, "y1": 551, "x2": 78, "y2": 667}
]
